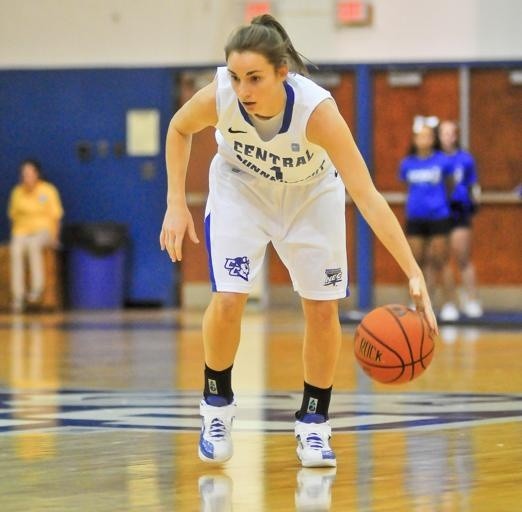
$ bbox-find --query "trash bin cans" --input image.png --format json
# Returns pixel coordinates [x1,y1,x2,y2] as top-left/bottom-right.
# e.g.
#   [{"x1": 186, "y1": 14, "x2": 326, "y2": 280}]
[{"x1": 61, "y1": 222, "x2": 131, "y2": 309}]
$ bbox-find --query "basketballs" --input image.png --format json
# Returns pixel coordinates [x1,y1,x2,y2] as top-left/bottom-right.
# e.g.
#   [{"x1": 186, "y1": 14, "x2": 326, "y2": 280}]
[{"x1": 353, "y1": 303, "x2": 436, "y2": 384}]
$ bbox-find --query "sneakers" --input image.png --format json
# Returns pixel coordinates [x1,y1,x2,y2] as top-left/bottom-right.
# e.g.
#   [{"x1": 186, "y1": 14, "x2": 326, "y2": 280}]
[
  {"x1": 197, "y1": 395, "x2": 238, "y2": 464},
  {"x1": 294, "y1": 409, "x2": 336, "y2": 468},
  {"x1": 438, "y1": 302, "x2": 460, "y2": 323},
  {"x1": 463, "y1": 299, "x2": 483, "y2": 319}
]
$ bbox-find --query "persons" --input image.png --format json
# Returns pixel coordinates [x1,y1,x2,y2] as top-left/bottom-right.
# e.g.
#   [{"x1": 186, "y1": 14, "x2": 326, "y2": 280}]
[
  {"x1": 159, "y1": 14, "x2": 439, "y2": 469},
  {"x1": 436, "y1": 120, "x2": 484, "y2": 322},
  {"x1": 398, "y1": 119, "x2": 456, "y2": 326},
  {"x1": 6, "y1": 159, "x2": 65, "y2": 314},
  {"x1": 198, "y1": 468, "x2": 339, "y2": 512}
]
